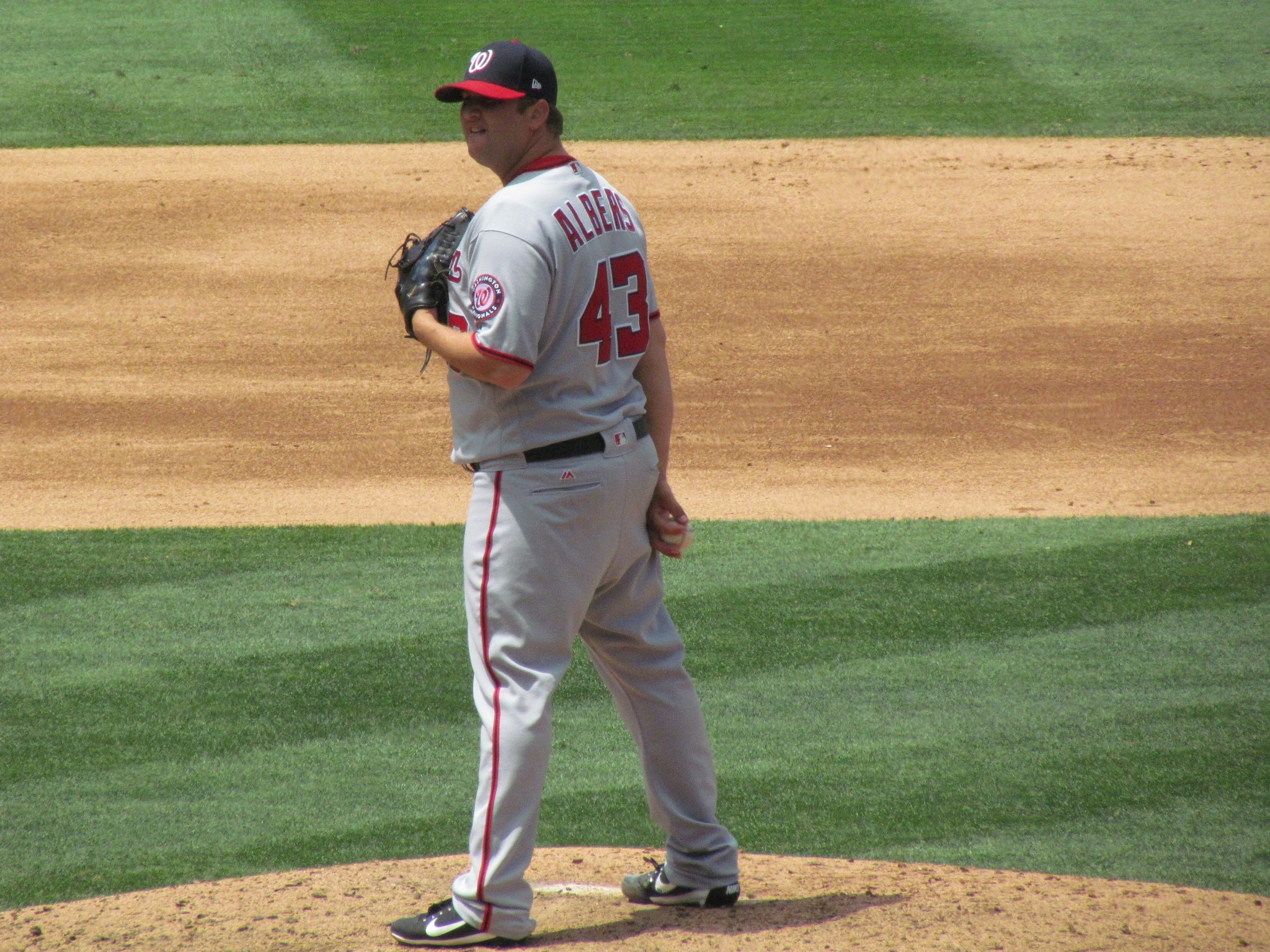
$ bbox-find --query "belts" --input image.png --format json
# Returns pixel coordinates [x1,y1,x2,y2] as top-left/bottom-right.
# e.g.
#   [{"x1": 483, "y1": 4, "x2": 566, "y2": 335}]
[{"x1": 472, "y1": 416, "x2": 648, "y2": 471}]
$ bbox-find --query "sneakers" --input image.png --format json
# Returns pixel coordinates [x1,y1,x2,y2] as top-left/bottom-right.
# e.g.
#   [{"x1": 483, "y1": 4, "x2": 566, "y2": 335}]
[
  {"x1": 622, "y1": 857, "x2": 740, "y2": 907},
  {"x1": 390, "y1": 897, "x2": 515, "y2": 947}
]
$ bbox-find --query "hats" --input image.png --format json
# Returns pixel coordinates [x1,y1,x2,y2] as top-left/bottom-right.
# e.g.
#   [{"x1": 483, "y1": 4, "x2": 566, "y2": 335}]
[{"x1": 434, "y1": 40, "x2": 557, "y2": 104}]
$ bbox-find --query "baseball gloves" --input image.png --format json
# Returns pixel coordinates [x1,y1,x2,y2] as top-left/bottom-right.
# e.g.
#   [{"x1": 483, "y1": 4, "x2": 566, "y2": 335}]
[{"x1": 393, "y1": 206, "x2": 477, "y2": 337}]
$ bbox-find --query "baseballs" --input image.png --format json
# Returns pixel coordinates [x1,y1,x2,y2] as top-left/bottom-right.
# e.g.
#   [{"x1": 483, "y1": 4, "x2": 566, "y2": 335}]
[{"x1": 661, "y1": 516, "x2": 695, "y2": 550}]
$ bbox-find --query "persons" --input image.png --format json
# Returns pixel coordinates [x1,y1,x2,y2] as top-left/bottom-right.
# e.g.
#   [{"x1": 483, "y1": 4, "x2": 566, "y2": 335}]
[{"x1": 383, "y1": 37, "x2": 742, "y2": 949}]
